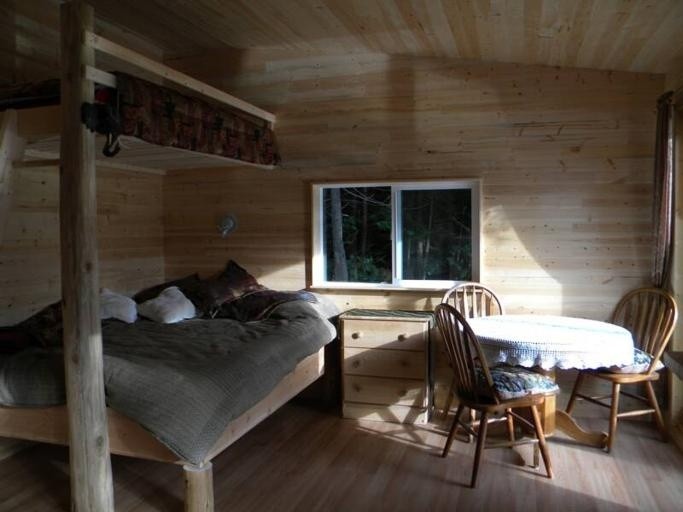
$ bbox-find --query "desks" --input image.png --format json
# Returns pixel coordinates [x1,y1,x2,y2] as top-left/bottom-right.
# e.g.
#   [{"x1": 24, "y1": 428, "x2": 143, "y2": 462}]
[{"x1": 452, "y1": 309, "x2": 640, "y2": 453}]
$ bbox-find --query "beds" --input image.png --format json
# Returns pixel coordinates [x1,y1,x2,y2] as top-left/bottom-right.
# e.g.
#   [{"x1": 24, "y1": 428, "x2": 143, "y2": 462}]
[
  {"x1": 0, "y1": 65, "x2": 289, "y2": 176},
  {"x1": 0, "y1": 269, "x2": 337, "y2": 512}
]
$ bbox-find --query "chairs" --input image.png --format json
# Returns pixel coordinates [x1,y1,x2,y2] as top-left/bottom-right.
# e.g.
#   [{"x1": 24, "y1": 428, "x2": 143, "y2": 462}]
[
  {"x1": 435, "y1": 282, "x2": 519, "y2": 424},
  {"x1": 566, "y1": 283, "x2": 681, "y2": 443},
  {"x1": 430, "y1": 303, "x2": 557, "y2": 490}
]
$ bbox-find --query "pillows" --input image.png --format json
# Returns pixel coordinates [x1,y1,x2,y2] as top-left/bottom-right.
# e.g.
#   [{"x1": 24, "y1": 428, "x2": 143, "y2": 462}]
[{"x1": 88, "y1": 259, "x2": 270, "y2": 326}]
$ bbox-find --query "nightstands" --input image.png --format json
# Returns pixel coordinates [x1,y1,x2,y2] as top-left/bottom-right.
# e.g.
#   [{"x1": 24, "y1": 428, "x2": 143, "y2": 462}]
[{"x1": 333, "y1": 306, "x2": 438, "y2": 426}]
